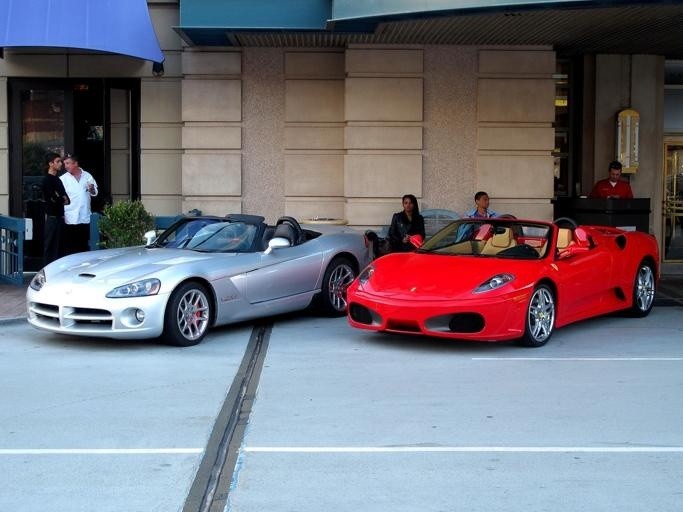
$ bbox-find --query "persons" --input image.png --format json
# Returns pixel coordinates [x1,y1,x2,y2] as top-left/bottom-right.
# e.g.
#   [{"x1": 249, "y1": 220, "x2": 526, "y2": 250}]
[
  {"x1": 454, "y1": 190, "x2": 500, "y2": 244},
  {"x1": 390, "y1": 194, "x2": 426, "y2": 253},
  {"x1": 587, "y1": 160, "x2": 634, "y2": 198},
  {"x1": 54, "y1": 153, "x2": 98, "y2": 253},
  {"x1": 41, "y1": 151, "x2": 70, "y2": 265}
]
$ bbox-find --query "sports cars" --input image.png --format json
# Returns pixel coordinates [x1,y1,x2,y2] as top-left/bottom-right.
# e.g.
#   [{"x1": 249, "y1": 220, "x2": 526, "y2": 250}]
[
  {"x1": 347, "y1": 218, "x2": 660, "y2": 348},
  {"x1": 25, "y1": 214, "x2": 372, "y2": 346}
]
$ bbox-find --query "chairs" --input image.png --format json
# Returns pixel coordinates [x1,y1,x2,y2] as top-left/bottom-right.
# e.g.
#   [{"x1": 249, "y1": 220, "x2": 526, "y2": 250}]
[
  {"x1": 179, "y1": 217, "x2": 302, "y2": 247},
  {"x1": 480, "y1": 227, "x2": 575, "y2": 260}
]
